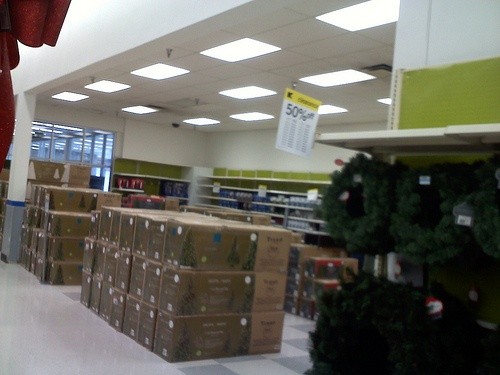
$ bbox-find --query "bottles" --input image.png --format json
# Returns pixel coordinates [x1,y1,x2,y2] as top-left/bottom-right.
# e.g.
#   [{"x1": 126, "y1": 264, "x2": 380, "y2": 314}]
[
  {"x1": 165, "y1": 182, "x2": 186, "y2": 197},
  {"x1": 218, "y1": 191, "x2": 319, "y2": 231},
  {"x1": 117, "y1": 177, "x2": 144, "y2": 190}
]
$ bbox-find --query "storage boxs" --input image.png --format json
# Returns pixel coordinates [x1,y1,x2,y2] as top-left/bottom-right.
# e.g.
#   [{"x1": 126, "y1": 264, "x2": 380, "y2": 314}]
[{"x1": 2, "y1": 154, "x2": 400, "y2": 362}]
